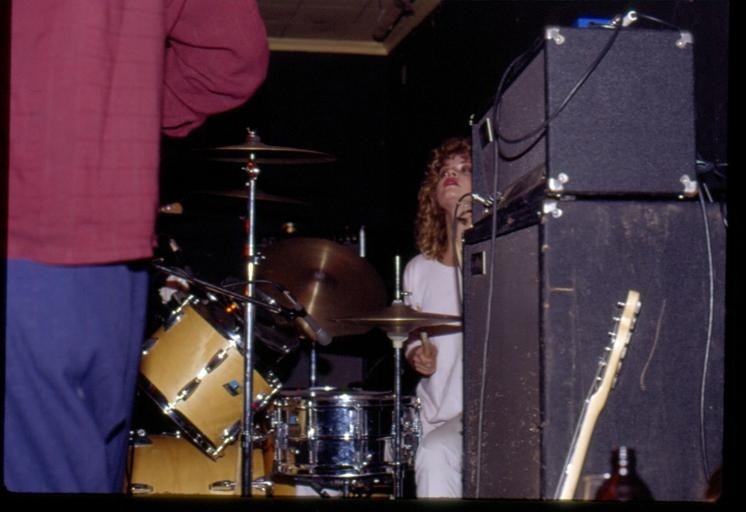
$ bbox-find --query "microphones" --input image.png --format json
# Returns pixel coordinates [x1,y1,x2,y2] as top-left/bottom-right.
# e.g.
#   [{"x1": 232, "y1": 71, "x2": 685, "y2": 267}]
[{"x1": 274, "y1": 282, "x2": 332, "y2": 345}]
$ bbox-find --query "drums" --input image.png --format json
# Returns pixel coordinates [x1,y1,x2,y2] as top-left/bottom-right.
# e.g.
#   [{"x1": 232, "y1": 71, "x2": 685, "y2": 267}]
[
  {"x1": 141, "y1": 294, "x2": 282, "y2": 461},
  {"x1": 256, "y1": 389, "x2": 396, "y2": 482}
]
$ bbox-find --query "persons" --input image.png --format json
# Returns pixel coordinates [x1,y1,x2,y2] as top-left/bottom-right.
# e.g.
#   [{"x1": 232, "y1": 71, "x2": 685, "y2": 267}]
[
  {"x1": 1, "y1": 0, "x2": 270, "y2": 491},
  {"x1": 403, "y1": 140, "x2": 474, "y2": 499}
]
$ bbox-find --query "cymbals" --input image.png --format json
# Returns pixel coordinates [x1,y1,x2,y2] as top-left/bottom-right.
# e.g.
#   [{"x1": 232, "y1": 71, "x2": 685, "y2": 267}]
[
  {"x1": 255, "y1": 239, "x2": 385, "y2": 341},
  {"x1": 212, "y1": 142, "x2": 335, "y2": 164},
  {"x1": 155, "y1": 261, "x2": 287, "y2": 313},
  {"x1": 327, "y1": 304, "x2": 462, "y2": 335}
]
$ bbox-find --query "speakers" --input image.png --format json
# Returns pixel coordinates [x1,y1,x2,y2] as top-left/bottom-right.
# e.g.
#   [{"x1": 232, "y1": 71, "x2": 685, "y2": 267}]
[
  {"x1": 469, "y1": 27, "x2": 698, "y2": 225},
  {"x1": 461, "y1": 200, "x2": 728, "y2": 512}
]
